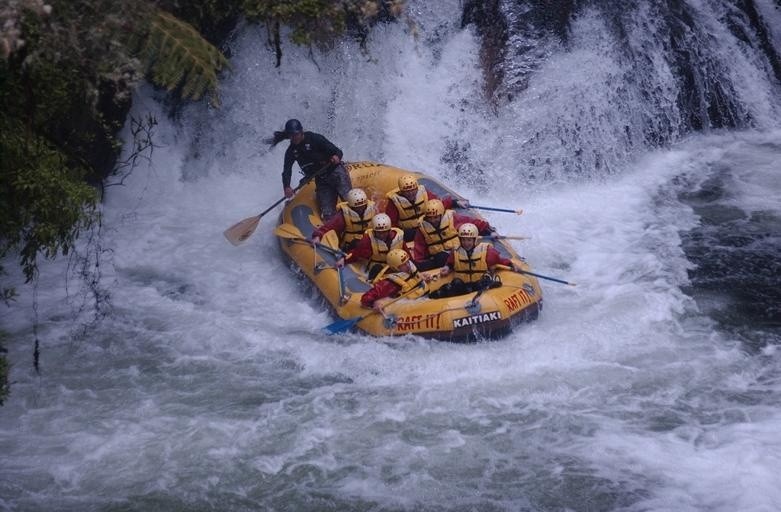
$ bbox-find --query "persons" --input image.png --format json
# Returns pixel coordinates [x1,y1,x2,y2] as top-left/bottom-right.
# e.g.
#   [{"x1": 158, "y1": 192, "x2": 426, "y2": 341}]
[
  {"x1": 310, "y1": 188, "x2": 380, "y2": 251},
  {"x1": 334, "y1": 213, "x2": 412, "y2": 273},
  {"x1": 361, "y1": 248, "x2": 432, "y2": 313},
  {"x1": 439, "y1": 223, "x2": 520, "y2": 296},
  {"x1": 385, "y1": 175, "x2": 470, "y2": 242},
  {"x1": 283, "y1": 120, "x2": 352, "y2": 224},
  {"x1": 413, "y1": 199, "x2": 500, "y2": 269}
]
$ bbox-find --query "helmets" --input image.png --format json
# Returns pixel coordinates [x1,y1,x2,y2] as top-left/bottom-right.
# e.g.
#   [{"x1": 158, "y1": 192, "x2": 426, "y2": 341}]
[
  {"x1": 346, "y1": 187, "x2": 369, "y2": 208},
  {"x1": 287, "y1": 119, "x2": 302, "y2": 137},
  {"x1": 371, "y1": 212, "x2": 392, "y2": 232},
  {"x1": 458, "y1": 222, "x2": 478, "y2": 246},
  {"x1": 397, "y1": 174, "x2": 418, "y2": 193},
  {"x1": 424, "y1": 200, "x2": 445, "y2": 216},
  {"x1": 385, "y1": 249, "x2": 410, "y2": 272}
]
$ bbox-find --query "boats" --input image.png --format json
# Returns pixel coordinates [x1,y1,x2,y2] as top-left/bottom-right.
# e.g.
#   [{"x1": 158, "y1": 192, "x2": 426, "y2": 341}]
[{"x1": 274, "y1": 163, "x2": 547, "y2": 345}]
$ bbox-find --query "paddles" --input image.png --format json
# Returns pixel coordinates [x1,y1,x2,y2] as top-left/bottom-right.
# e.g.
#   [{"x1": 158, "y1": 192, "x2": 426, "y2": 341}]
[
  {"x1": 272, "y1": 224, "x2": 348, "y2": 259},
  {"x1": 223, "y1": 161, "x2": 330, "y2": 246},
  {"x1": 321, "y1": 286, "x2": 422, "y2": 336}
]
